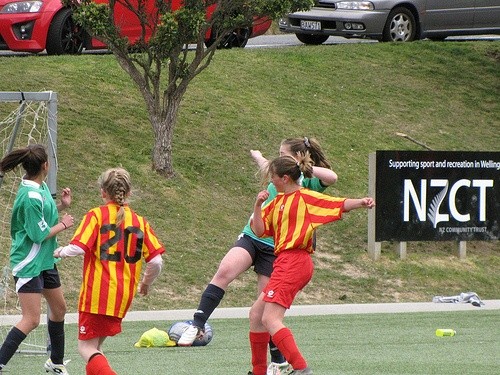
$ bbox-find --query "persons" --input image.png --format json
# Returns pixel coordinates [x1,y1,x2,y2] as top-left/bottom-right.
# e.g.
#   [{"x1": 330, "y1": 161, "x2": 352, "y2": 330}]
[
  {"x1": 1, "y1": 145, "x2": 77, "y2": 374},
  {"x1": 54, "y1": 168, "x2": 166, "y2": 375},
  {"x1": 246, "y1": 155, "x2": 375, "y2": 375},
  {"x1": 177, "y1": 135, "x2": 336, "y2": 374}
]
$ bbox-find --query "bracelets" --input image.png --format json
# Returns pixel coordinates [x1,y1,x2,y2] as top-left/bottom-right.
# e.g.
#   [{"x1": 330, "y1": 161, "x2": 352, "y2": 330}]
[{"x1": 61, "y1": 220, "x2": 67, "y2": 230}]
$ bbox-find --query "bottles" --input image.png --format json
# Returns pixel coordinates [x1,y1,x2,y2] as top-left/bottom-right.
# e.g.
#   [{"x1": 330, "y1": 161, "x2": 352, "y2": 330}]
[{"x1": 436, "y1": 329, "x2": 457, "y2": 337}]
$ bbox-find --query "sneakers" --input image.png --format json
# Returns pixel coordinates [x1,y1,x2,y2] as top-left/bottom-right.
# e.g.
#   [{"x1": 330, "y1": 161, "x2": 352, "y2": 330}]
[
  {"x1": 44, "y1": 358, "x2": 70, "y2": 374},
  {"x1": 265, "y1": 361, "x2": 295, "y2": 375},
  {"x1": 288, "y1": 365, "x2": 314, "y2": 375},
  {"x1": 177, "y1": 322, "x2": 203, "y2": 346}
]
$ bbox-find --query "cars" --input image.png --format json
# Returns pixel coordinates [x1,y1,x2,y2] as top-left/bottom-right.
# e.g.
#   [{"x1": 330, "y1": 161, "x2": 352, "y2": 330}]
[
  {"x1": 0, "y1": 0, "x2": 273, "y2": 56},
  {"x1": 278, "y1": 0, "x2": 500, "y2": 45}
]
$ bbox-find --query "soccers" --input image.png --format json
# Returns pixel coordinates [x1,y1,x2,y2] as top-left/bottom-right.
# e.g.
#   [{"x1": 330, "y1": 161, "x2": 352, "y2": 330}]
[{"x1": 168, "y1": 320, "x2": 213, "y2": 346}]
